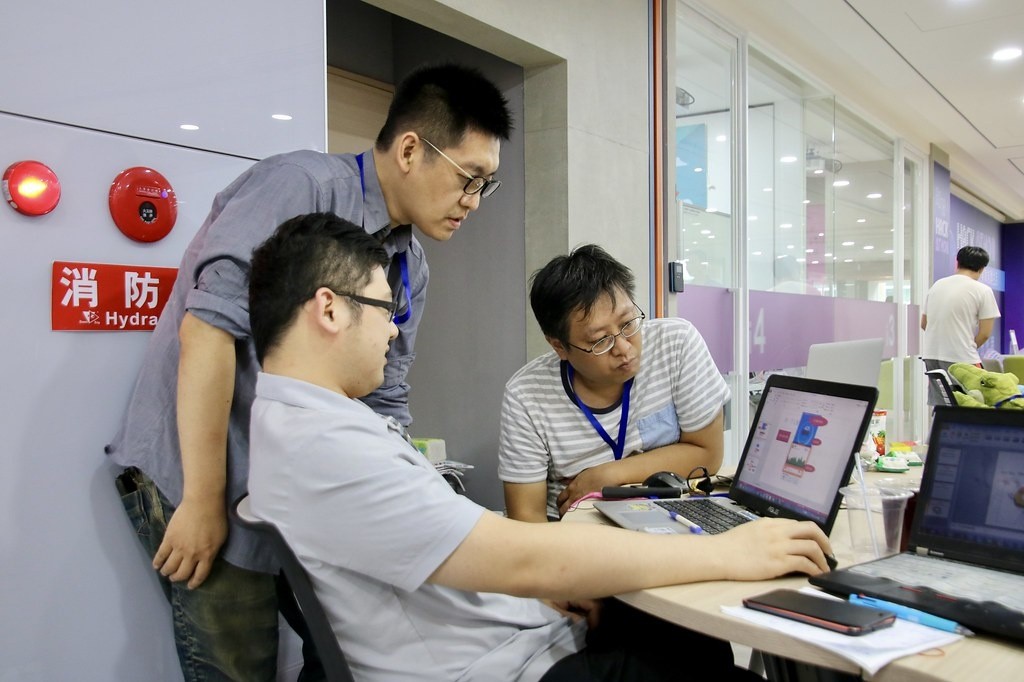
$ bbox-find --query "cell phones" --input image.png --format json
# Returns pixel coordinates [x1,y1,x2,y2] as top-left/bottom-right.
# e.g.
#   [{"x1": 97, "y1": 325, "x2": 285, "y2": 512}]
[{"x1": 742, "y1": 589, "x2": 897, "y2": 635}]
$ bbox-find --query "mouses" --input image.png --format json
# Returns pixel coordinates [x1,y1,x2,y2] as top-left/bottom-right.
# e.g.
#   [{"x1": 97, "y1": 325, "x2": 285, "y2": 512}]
[
  {"x1": 782, "y1": 554, "x2": 839, "y2": 578},
  {"x1": 642, "y1": 471, "x2": 691, "y2": 494}
]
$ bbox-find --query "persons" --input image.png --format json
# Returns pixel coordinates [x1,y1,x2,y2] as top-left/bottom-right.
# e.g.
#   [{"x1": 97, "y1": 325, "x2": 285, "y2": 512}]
[
  {"x1": 98, "y1": 62, "x2": 514, "y2": 682},
  {"x1": 917, "y1": 247, "x2": 1003, "y2": 402},
  {"x1": 496, "y1": 243, "x2": 738, "y2": 667},
  {"x1": 246, "y1": 212, "x2": 837, "y2": 682}
]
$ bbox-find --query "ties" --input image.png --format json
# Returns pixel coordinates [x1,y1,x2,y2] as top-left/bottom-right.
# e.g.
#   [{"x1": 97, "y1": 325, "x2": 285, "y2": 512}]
[{"x1": 382, "y1": 414, "x2": 421, "y2": 455}]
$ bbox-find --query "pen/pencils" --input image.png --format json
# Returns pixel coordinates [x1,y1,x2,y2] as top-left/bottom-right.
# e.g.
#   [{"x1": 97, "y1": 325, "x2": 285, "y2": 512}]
[
  {"x1": 669, "y1": 511, "x2": 702, "y2": 534},
  {"x1": 850, "y1": 594, "x2": 976, "y2": 638}
]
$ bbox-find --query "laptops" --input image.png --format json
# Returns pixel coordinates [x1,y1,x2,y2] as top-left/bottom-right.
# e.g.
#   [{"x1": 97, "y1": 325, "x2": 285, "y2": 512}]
[
  {"x1": 593, "y1": 373, "x2": 880, "y2": 536},
  {"x1": 805, "y1": 337, "x2": 887, "y2": 386},
  {"x1": 808, "y1": 406, "x2": 1024, "y2": 646}
]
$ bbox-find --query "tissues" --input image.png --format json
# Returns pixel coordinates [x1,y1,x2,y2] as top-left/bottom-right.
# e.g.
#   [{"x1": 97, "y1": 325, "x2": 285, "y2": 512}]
[{"x1": 411, "y1": 438, "x2": 446, "y2": 463}]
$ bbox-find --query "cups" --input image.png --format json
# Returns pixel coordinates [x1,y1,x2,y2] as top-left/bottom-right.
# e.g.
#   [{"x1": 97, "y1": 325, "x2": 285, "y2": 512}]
[
  {"x1": 874, "y1": 477, "x2": 920, "y2": 552},
  {"x1": 839, "y1": 484, "x2": 914, "y2": 561}
]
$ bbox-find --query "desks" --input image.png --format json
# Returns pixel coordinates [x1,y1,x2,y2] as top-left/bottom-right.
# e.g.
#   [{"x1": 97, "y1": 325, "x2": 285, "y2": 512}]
[{"x1": 558, "y1": 445, "x2": 1024, "y2": 682}]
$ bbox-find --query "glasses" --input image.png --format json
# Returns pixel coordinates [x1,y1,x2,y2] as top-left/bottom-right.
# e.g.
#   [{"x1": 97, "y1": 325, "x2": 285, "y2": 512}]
[
  {"x1": 300, "y1": 288, "x2": 398, "y2": 323},
  {"x1": 419, "y1": 137, "x2": 502, "y2": 199},
  {"x1": 558, "y1": 301, "x2": 645, "y2": 356}
]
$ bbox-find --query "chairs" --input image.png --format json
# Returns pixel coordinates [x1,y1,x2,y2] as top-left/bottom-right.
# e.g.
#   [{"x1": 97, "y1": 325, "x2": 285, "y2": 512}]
[{"x1": 220, "y1": 490, "x2": 355, "y2": 682}]
